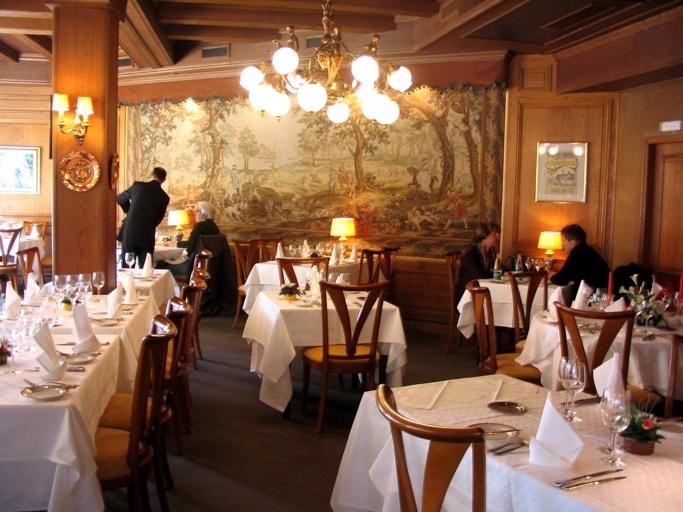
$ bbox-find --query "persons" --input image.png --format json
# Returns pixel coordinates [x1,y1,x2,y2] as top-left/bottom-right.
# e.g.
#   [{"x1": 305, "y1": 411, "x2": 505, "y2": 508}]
[
  {"x1": 117, "y1": 167, "x2": 169, "y2": 267},
  {"x1": 551, "y1": 223, "x2": 609, "y2": 301},
  {"x1": 416, "y1": 167, "x2": 434, "y2": 194},
  {"x1": 154, "y1": 201, "x2": 220, "y2": 275},
  {"x1": 456, "y1": 221, "x2": 514, "y2": 353},
  {"x1": 439, "y1": 186, "x2": 466, "y2": 231}
]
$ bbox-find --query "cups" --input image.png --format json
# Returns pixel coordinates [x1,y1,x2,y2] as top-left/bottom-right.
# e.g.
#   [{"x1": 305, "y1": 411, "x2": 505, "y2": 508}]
[
  {"x1": 302, "y1": 270, "x2": 338, "y2": 284},
  {"x1": 661, "y1": 291, "x2": 670, "y2": 311},
  {"x1": 524, "y1": 255, "x2": 546, "y2": 272}
]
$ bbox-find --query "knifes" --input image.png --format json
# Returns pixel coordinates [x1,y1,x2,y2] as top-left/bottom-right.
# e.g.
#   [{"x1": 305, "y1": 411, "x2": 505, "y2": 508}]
[
  {"x1": 560, "y1": 395, "x2": 601, "y2": 406},
  {"x1": 551, "y1": 468, "x2": 629, "y2": 491}
]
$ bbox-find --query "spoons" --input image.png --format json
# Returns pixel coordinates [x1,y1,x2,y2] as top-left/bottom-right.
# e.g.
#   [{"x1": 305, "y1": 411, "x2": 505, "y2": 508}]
[{"x1": 494, "y1": 437, "x2": 534, "y2": 456}]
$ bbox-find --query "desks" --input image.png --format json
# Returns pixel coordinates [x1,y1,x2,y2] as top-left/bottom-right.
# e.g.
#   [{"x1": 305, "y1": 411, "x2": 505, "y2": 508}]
[
  {"x1": 1, "y1": 267, "x2": 172, "y2": 512},
  {"x1": 148, "y1": 242, "x2": 187, "y2": 270},
  {"x1": 256, "y1": 255, "x2": 398, "y2": 400},
  {"x1": 475, "y1": 267, "x2": 574, "y2": 355},
  {"x1": 362, "y1": 372, "x2": 681, "y2": 512},
  {"x1": 534, "y1": 309, "x2": 682, "y2": 415}
]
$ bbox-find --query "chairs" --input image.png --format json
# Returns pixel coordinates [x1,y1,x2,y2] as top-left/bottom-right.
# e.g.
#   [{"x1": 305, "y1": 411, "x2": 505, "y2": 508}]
[
  {"x1": 659, "y1": 333, "x2": 683, "y2": 418},
  {"x1": 509, "y1": 269, "x2": 552, "y2": 349},
  {"x1": 439, "y1": 249, "x2": 477, "y2": 355},
  {"x1": 0, "y1": 227, "x2": 25, "y2": 298},
  {"x1": 15, "y1": 247, "x2": 48, "y2": 291},
  {"x1": 546, "y1": 301, "x2": 638, "y2": 400},
  {"x1": 228, "y1": 237, "x2": 284, "y2": 333},
  {"x1": 90, "y1": 247, "x2": 213, "y2": 510},
  {"x1": 356, "y1": 247, "x2": 404, "y2": 299},
  {"x1": 605, "y1": 260, "x2": 654, "y2": 300},
  {"x1": 293, "y1": 277, "x2": 397, "y2": 436},
  {"x1": 465, "y1": 277, "x2": 543, "y2": 382},
  {"x1": 374, "y1": 380, "x2": 488, "y2": 512}
]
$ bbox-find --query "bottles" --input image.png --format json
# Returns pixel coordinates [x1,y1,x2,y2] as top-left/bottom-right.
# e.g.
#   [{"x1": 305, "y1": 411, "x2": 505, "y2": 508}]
[
  {"x1": 492, "y1": 253, "x2": 502, "y2": 281},
  {"x1": 514, "y1": 254, "x2": 522, "y2": 281},
  {"x1": 31, "y1": 223, "x2": 39, "y2": 239}
]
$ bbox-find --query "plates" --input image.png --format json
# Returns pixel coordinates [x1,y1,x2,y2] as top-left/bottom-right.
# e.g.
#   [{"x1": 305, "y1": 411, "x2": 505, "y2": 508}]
[
  {"x1": 121, "y1": 303, "x2": 134, "y2": 312},
  {"x1": 287, "y1": 300, "x2": 312, "y2": 307},
  {"x1": 487, "y1": 401, "x2": 528, "y2": 416},
  {"x1": 95, "y1": 316, "x2": 117, "y2": 328},
  {"x1": 468, "y1": 421, "x2": 520, "y2": 442},
  {"x1": 21, "y1": 381, "x2": 69, "y2": 404}
]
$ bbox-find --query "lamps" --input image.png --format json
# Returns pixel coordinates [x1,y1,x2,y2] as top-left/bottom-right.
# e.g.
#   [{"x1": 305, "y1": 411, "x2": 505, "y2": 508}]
[
  {"x1": 168, "y1": 210, "x2": 190, "y2": 232},
  {"x1": 330, "y1": 218, "x2": 356, "y2": 241},
  {"x1": 240, "y1": 0, "x2": 413, "y2": 125},
  {"x1": 52, "y1": 92, "x2": 93, "y2": 135}
]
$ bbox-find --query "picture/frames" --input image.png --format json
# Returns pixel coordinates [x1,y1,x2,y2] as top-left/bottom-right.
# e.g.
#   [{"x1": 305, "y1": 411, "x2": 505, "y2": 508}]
[
  {"x1": 535, "y1": 142, "x2": 588, "y2": 204},
  {"x1": 1, "y1": 145, "x2": 41, "y2": 195}
]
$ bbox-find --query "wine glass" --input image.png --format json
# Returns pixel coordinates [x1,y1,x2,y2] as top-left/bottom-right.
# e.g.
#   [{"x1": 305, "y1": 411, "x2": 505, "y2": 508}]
[
  {"x1": 591, "y1": 287, "x2": 656, "y2": 338},
  {"x1": 1, "y1": 270, "x2": 104, "y2": 377},
  {"x1": 124, "y1": 251, "x2": 136, "y2": 274},
  {"x1": 555, "y1": 355, "x2": 588, "y2": 423},
  {"x1": 598, "y1": 386, "x2": 629, "y2": 468},
  {"x1": 286, "y1": 240, "x2": 347, "y2": 260},
  {"x1": 672, "y1": 290, "x2": 681, "y2": 319}
]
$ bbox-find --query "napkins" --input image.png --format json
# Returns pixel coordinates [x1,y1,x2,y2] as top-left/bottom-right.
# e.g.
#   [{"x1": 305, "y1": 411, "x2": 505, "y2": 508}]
[
  {"x1": 593, "y1": 352, "x2": 625, "y2": 407},
  {"x1": 528, "y1": 390, "x2": 582, "y2": 469}
]
